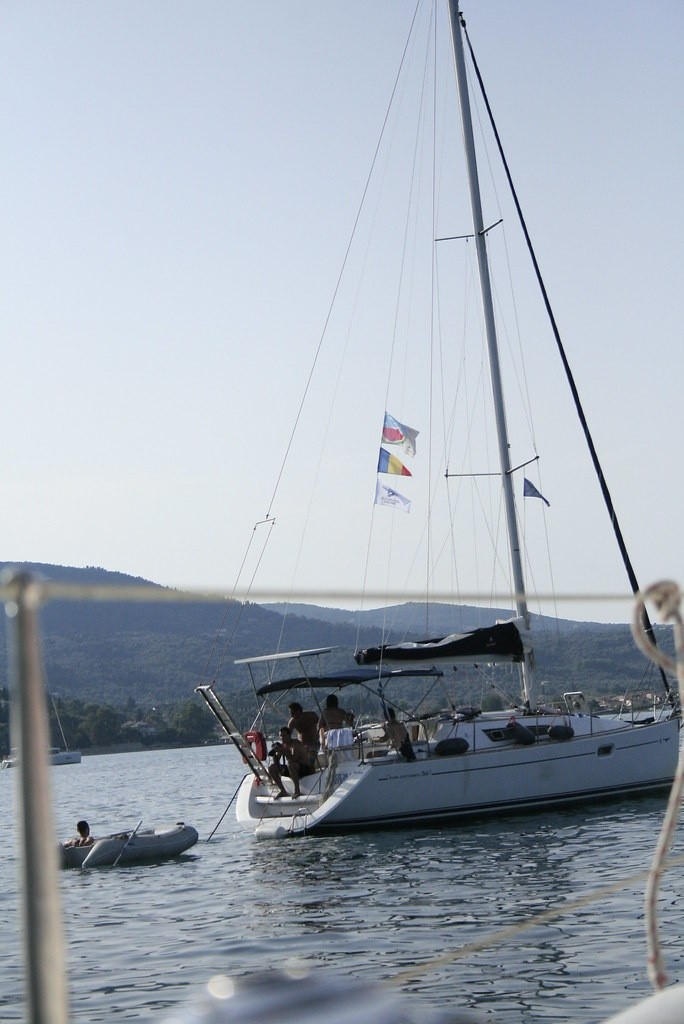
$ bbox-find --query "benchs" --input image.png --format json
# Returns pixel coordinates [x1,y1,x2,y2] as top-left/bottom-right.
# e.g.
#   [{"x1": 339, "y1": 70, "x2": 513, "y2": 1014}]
[{"x1": 314, "y1": 742, "x2": 388, "y2": 769}]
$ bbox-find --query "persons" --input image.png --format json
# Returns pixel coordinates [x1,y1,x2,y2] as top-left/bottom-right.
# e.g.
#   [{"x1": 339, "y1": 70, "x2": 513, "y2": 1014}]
[
  {"x1": 372, "y1": 707, "x2": 408, "y2": 751},
  {"x1": 287, "y1": 702, "x2": 320, "y2": 763},
  {"x1": 268, "y1": 727, "x2": 311, "y2": 801},
  {"x1": 318, "y1": 694, "x2": 355, "y2": 748},
  {"x1": 64, "y1": 820, "x2": 94, "y2": 848}
]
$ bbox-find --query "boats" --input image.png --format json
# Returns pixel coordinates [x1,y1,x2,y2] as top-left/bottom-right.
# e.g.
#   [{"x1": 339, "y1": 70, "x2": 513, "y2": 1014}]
[{"x1": 55, "y1": 819, "x2": 201, "y2": 871}]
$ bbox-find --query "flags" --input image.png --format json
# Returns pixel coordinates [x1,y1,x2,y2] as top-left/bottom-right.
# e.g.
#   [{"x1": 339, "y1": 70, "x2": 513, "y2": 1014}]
[
  {"x1": 374, "y1": 479, "x2": 411, "y2": 514},
  {"x1": 523, "y1": 477, "x2": 550, "y2": 507},
  {"x1": 381, "y1": 411, "x2": 420, "y2": 458}
]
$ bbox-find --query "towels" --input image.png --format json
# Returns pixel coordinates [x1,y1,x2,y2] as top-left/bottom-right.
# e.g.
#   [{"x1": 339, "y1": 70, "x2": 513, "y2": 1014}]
[
  {"x1": 418, "y1": 717, "x2": 439, "y2": 741},
  {"x1": 324, "y1": 727, "x2": 353, "y2": 750}
]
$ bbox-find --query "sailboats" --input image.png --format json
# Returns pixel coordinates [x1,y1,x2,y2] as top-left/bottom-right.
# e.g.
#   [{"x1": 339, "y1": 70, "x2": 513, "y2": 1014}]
[
  {"x1": 152, "y1": 0, "x2": 684, "y2": 842},
  {"x1": 0, "y1": 665, "x2": 82, "y2": 770}
]
